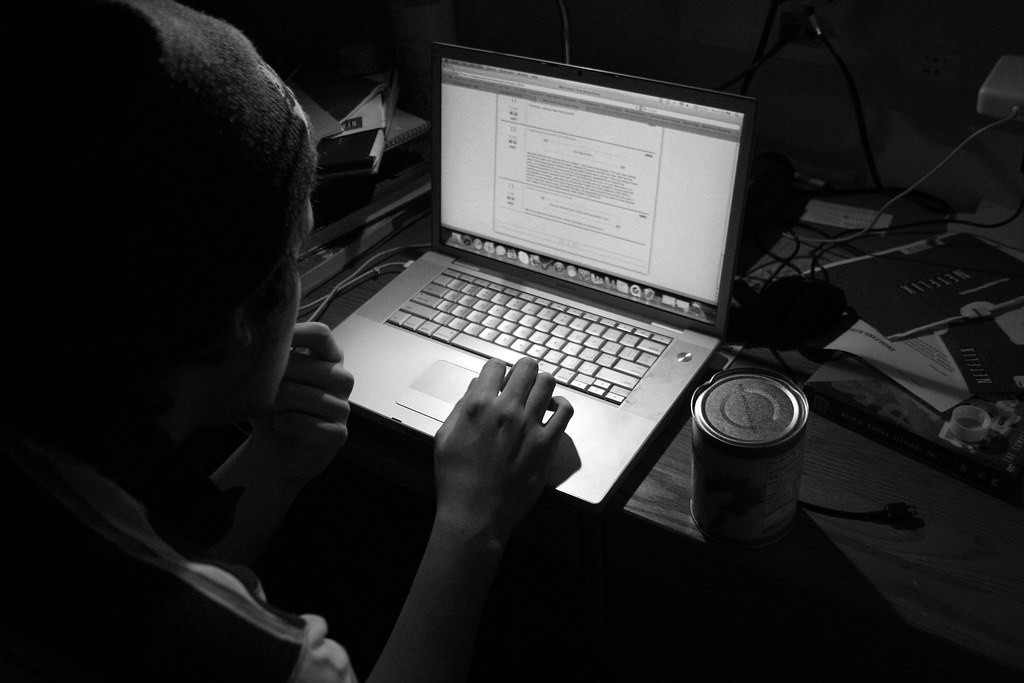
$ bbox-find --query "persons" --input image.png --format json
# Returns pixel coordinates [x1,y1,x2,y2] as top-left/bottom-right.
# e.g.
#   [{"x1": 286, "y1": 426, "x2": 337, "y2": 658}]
[{"x1": 0, "y1": 1, "x2": 575, "y2": 683}]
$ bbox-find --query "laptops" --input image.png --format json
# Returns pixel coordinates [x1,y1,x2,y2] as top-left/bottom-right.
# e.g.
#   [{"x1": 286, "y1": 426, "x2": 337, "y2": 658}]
[{"x1": 330, "y1": 41, "x2": 758, "y2": 504}]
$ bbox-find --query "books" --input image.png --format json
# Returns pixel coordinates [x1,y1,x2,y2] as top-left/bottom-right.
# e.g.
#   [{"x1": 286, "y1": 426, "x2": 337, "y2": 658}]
[{"x1": 285, "y1": 61, "x2": 433, "y2": 302}]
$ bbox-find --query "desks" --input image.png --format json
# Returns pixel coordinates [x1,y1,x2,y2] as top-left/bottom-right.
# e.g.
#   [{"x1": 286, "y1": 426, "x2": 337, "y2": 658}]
[{"x1": 302, "y1": 207, "x2": 1023, "y2": 683}]
